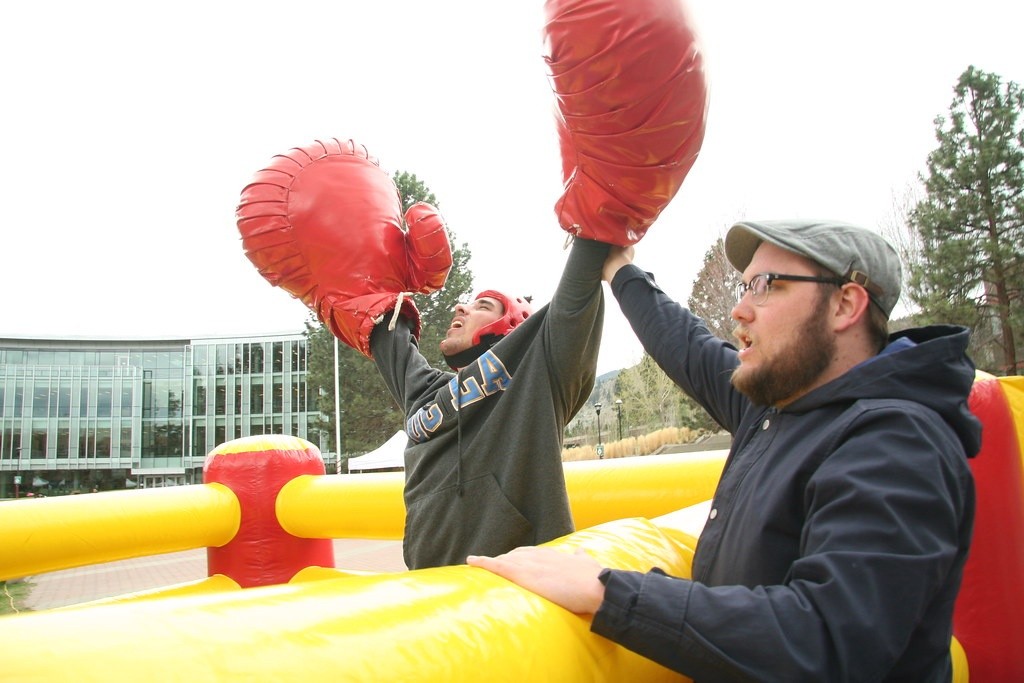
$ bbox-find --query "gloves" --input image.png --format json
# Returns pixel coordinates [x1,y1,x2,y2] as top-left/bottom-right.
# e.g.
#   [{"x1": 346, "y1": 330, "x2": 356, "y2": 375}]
[
  {"x1": 234, "y1": 137, "x2": 454, "y2": 356},
  {"x1": 544, "y1": 0, "x2": 709, "y2": 247}
]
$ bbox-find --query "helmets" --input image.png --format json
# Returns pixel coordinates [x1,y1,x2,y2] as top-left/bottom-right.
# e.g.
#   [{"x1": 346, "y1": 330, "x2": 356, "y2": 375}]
[{"x1": 445, "y1": 289, "x2": 535, "y2": 371}]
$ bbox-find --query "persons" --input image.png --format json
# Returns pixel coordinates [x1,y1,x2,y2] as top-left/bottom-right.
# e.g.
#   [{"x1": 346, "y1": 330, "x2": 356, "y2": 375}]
[
  {"x1": 465, "y1": 220, "x2": 981, "y2": 683},
  {"x1": 235, "y1": 2, "x2": 708, "y2": 572}
]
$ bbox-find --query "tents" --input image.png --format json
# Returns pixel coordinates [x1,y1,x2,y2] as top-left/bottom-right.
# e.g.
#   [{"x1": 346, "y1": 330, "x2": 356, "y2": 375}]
[{"x1": 346, "y1": 429, "x2": 411, "y2": 476}]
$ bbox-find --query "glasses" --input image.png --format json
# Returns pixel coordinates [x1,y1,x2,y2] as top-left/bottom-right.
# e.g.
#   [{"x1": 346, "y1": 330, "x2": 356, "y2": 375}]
[{"x1": 734, "y1": 273, "x2": 847, "y2": 307}]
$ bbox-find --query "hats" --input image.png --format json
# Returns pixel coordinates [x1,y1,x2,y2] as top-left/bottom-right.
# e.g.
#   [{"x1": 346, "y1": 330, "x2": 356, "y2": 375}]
[{"x1": 725, "y1": 220, "x2": 903, "y2": 320}]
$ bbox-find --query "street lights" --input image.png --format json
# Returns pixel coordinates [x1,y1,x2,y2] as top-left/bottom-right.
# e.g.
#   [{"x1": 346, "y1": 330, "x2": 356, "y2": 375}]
[
  {"x1": 595, "y1": 403, "x2": 605, "y2": 460},
  {"x1": 12, "y1": 447, "x2": 25, "y2": 499},
  {"x1": 616, "y1": 399, "x2": 625, "y2": 441}
]
